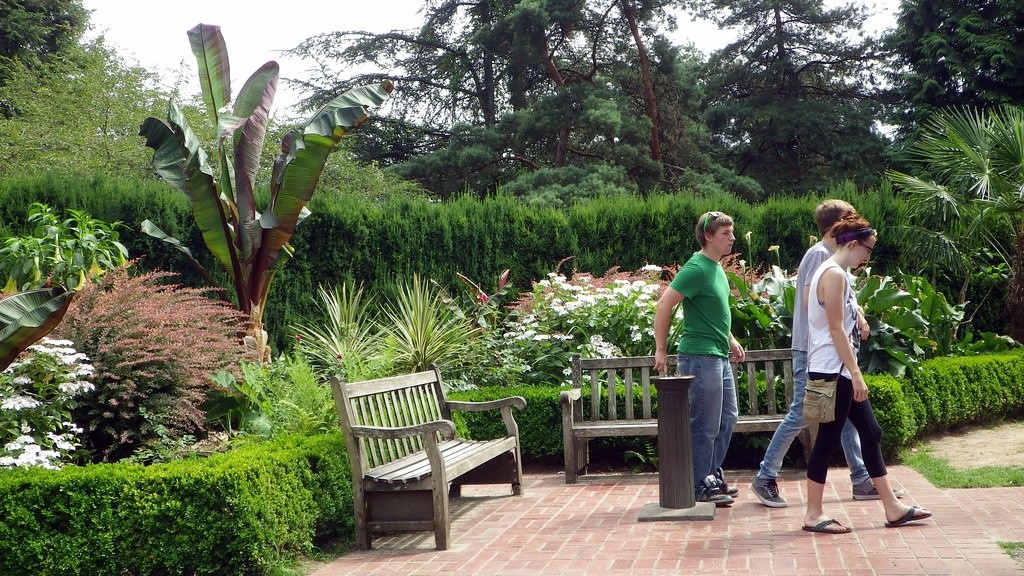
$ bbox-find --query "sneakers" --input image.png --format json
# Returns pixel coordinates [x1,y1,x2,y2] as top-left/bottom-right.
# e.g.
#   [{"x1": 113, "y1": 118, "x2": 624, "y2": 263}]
[
  {"x1": 751, "y1": 475, "x2": 787, "y2": 508},
  {"x1": 709, "y1": 467, "x2": 739, "y2": 497},
  {"x1": 695, "y1": 474, "x2": 734, "y2": 505},
  {"x1": 853, "y1": 477, "x2": 904, "y2": 500}
]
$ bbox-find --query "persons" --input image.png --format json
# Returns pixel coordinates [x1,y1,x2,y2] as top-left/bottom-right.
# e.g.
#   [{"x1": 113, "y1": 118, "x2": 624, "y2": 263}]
[
  {"x1": 802, "y1": 211, "x2": 932, "y2": 534},
  {"x1": 652, "y1": 211, "x2": 745, "y2": 503},
  {"x1": 751, "y1": 198, "x2": 903, "y2": 506}
]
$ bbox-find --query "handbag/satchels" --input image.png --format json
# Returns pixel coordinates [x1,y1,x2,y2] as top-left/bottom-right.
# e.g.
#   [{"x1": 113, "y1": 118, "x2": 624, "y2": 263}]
[{"x1": 803, "y1": 379, "x2": 837, "y2": 423}]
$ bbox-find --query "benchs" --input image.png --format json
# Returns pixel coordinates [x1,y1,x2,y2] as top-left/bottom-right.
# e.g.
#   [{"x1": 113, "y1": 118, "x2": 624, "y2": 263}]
[
  {"x1": 330, "y1": 363, "x2": 527, "y2": 551},
  {"x1": 559, "y1": 348, "x2": 811, "y2": 484}
]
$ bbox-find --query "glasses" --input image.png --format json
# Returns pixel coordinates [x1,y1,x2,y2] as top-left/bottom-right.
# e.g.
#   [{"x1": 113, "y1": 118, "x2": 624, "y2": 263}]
[
  {"x1": 704, "y1": 212, "x2": 724, "y2": 232},
  {"x1": 847, "y1": 239, "x2": 873, "y2": 255}
]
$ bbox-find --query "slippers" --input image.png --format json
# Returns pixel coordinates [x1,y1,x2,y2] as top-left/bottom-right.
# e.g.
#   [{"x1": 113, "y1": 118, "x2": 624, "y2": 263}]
[
  {"x1": 885, "y1": 506, "x2": 932, "y2": 527},
  {"x1": 802, "y1": 519, "x2": 851, "y2": 533}
]
